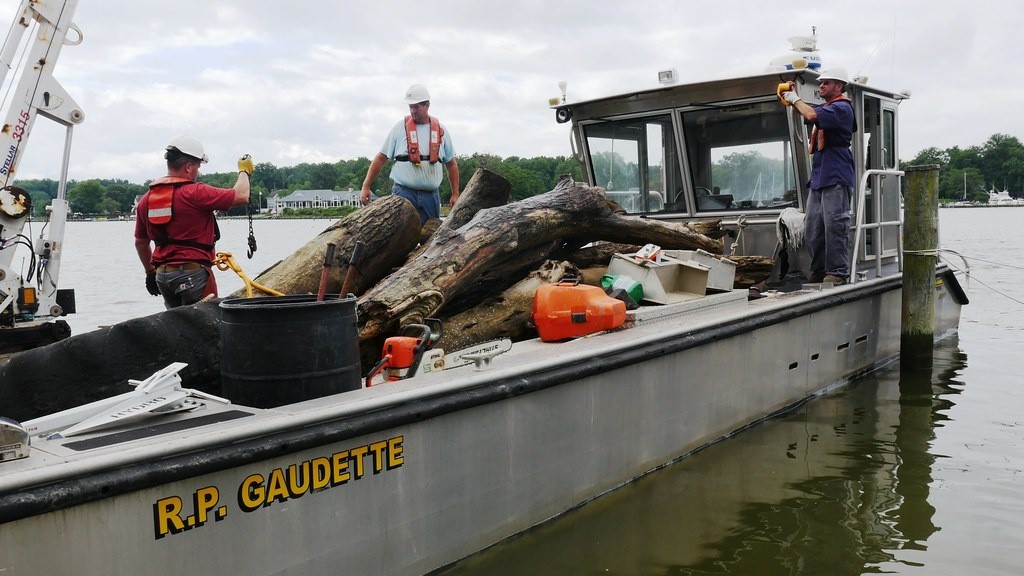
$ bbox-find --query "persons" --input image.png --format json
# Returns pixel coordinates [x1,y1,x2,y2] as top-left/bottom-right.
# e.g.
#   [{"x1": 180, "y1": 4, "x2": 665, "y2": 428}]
[
  {"x1": 361, "y1": 85, "x2": 459, "y2": 227},
  {"x1": 135, "y1": 135, "x2": 254, "y2": 311},
  {"x1": 782, "y1": 66, "x2": 857, "y2": 286}
]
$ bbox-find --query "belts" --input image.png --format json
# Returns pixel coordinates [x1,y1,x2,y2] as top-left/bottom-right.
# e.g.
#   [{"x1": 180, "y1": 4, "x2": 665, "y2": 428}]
[{"x1": 156, "y1": 264, "x2": 201, "y2": 271}]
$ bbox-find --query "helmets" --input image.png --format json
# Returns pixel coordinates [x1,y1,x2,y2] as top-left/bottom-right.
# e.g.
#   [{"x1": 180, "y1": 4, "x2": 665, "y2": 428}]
[
  {"x1": 165, "y1": 137, "x2": 209, "y2": 164},
  {"x1": 406, "y1": 84, "x2": 430, "y2": 104},
  {"x1": 815, "y1": 67, "x2": 849, "y2": 86}
]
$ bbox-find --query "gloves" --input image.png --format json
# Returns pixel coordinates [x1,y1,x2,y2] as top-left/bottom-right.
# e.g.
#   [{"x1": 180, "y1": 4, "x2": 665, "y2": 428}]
[
  {"x1": 781, "y1": 86, "x2": 802, "y2": 105},
  {"x1": 238, "y1": 156, "x2": 255, "y2": 177},
  {"x1": 145, "y1": 268, "x2": 161, "y2": 296}
]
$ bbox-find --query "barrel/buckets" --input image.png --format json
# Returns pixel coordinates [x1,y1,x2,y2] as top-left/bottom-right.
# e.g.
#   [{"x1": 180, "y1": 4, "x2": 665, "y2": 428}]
[{"x1": 219, "y1": 292, "x2": 362, "y2": 410}]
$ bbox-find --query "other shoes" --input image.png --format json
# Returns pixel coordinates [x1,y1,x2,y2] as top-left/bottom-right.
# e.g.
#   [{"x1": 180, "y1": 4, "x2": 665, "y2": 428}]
[
  {"x1": 823, "y1": 274, "x2": 846, "y2": 285},
  {"x1": 807, "y1": 273, "x2": 826, "y2": 282}
]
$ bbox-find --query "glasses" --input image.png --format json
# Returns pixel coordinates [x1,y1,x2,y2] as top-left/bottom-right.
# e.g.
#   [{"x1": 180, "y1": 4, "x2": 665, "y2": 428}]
[{"x1": 819, "y1": 80, "x2": 838, "y2": 85}]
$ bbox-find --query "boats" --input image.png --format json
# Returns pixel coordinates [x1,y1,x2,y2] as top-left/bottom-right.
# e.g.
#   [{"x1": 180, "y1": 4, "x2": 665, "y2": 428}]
[{"x1": 0, "y1": 26, "x2": 972, "y2": 576}]
